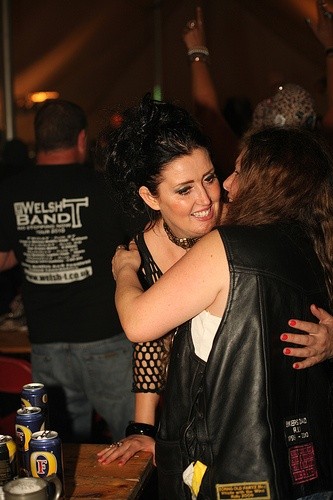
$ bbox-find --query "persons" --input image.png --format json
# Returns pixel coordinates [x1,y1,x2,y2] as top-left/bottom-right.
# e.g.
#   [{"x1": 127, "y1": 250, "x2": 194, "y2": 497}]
[
  {"x1": 112, "y1": 125, "x2": 333, "y2": 500},
  {"x1": 0, "y1": 7, "x2": 333, "y2": 469}
]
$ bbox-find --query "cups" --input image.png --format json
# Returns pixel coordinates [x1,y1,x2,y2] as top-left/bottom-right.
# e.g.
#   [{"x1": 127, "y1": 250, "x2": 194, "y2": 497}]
[{"x1": 2, "y1": 473, "x2": 62, "y2": 500}]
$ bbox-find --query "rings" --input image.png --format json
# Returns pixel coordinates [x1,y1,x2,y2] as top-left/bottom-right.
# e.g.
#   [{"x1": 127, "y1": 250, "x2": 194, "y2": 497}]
[
  {"x1": 117, "y1": 442, "x2": 123, "y2": 445},
  {"x1": 189, "y1": 22, "x2": 199, "y2": 30},
  {"x1": 110, "y1": 444, "x2": 115, "y2": 447}
]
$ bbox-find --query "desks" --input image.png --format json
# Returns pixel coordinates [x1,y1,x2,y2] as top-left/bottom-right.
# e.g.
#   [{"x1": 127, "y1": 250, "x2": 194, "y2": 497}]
[{"x1": 58, "y1": 441, "x2": 153, "y2": 500}]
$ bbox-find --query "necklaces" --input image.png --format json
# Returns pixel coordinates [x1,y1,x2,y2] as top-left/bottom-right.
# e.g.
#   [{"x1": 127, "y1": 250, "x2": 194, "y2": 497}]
[{"x1": 163, "y1": 222, "x2": 198, "y2": 248}]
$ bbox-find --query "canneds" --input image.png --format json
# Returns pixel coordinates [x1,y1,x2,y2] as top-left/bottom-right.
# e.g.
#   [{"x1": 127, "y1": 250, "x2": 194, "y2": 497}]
[
  {"x1": 21, "y1": 382, "x2": 48, "y2": 431},
  {"x1": 0, "y1": 435, "x2": 19, "y2": 489},
  {"x1": 28, "y1": 430, "x2": 66, "y2": 500},
  {"x1": 15, "y1": 406, "x2": 45, "y2": 478}
]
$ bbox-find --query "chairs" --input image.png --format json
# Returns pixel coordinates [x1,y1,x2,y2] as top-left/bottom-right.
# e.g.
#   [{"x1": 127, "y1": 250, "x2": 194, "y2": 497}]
[{"x1": 0, "y1": 357, "x2": 35, "y2": 435}]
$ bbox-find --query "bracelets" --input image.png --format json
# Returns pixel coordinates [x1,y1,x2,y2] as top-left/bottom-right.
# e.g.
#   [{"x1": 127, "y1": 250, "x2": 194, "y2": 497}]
[
  {"x1": 323, "y1": 48, "x2": 333, "y2": 57},
  {"x1": 125, "y1": 423, "x2": 156, "y2": 439},
  {"x1": 187, "y1": 50, "x2": 209, "y2": 57},
  {"x1": 189, "y1": 54, "x2": 211, "y2": 67},
  {"x1": 186, "y1": 46, "x2": 209, "y2": 52}
]
